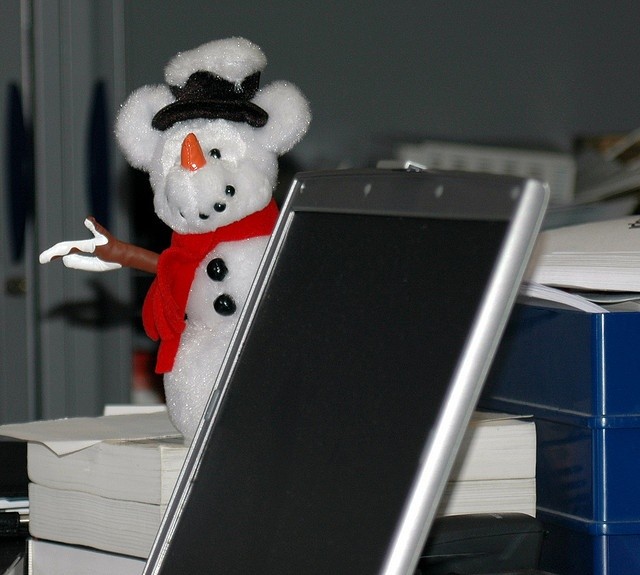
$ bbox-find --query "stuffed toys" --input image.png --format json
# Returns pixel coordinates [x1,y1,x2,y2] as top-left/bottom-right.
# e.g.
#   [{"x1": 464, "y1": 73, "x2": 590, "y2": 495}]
[{"x1": 38, "y1": 33, "x2": 313, "y2": 442}]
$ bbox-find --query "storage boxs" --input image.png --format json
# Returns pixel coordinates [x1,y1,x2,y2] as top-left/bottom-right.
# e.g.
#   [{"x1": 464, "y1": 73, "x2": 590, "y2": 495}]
[{"x1": 480, "y1": 301, "x2": 639, "y2": 574}]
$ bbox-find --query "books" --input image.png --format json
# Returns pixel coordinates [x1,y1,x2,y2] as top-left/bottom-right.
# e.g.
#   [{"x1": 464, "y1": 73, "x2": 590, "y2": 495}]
[
  {"x1": 517, "y1": 215, "x2": 640, "y2": 312},
  {"x1": 29, "y1": 478, "x2": 536, "y2": 561},
  {"x1": 25, "y1": 537, "x2": 148, "y2": 574},
  {"x1": 26, "y1": 410, "x2": 537, "y2": 507}
]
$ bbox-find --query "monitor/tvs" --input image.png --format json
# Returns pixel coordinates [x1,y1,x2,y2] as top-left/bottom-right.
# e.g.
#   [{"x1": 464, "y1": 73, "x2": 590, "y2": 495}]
[{"x1": 143, "y1": 160, "x2": 551, "y2": 574}]
